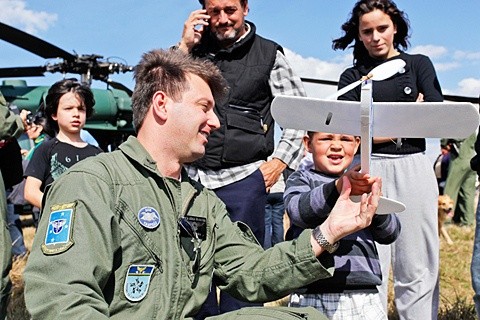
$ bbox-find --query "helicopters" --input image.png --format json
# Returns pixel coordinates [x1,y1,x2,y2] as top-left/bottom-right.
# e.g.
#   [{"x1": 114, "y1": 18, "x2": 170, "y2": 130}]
[{"x1": 0, "y1": 21, "x2": 136, "y2": 152}]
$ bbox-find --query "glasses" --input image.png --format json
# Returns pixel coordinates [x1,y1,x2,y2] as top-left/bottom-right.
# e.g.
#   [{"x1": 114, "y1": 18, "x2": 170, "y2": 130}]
[{"x1": 175, "y1": 216, "x2": 201, "y2": 273}]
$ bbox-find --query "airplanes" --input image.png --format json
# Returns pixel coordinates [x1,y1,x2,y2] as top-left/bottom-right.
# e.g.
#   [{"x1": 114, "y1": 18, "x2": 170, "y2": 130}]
[{"x1": 270, "y1": 59, "x2": 480, "y2": 214}]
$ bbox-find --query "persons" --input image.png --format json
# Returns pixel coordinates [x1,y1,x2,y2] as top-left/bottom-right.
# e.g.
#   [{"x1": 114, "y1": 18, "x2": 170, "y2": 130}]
[
  {"x1": 331, "y1": 0, "x2": 446, "y2": 320},
  {"x1": 175, "y1": 0, "x2": 310, "y2": 319},
  {"x1": 281, "y1": 130, "x2": 403, "y2": 320},
  {"x1": 0, "y1": 78, "x2": 103, "y2": 320},
  {"x1": 437, "y1": 128, "x2": 480, "y2": 318},
  {"x1": 23, "y1": 47, "x2": 382, "y2": 320},
  {"x1": 263, "y1": 174, "x2": 289, "y2": 250}
]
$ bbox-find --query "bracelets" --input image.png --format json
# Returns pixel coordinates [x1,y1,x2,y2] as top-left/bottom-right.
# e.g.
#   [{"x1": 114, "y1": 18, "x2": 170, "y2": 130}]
[{"x1": 313, "y1": 224, "x2": 341, "y2": 254}]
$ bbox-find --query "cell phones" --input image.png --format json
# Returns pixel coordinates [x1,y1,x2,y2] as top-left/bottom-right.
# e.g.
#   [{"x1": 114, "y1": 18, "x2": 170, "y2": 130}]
[{"x1": 203, "y1": 12, "x2": 208, "y2": 31}]
